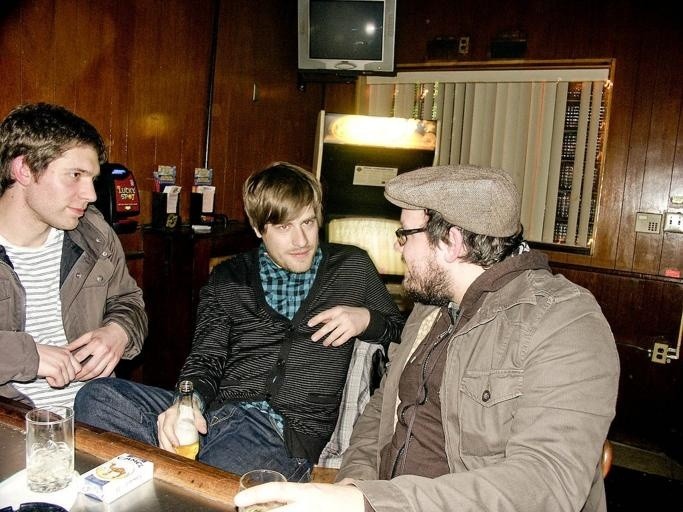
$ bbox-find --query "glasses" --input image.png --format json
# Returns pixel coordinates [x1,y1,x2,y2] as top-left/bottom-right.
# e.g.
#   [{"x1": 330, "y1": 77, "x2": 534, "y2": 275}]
[{"x1": 395, "y1": 228, "x2": 427, "y2": 246}]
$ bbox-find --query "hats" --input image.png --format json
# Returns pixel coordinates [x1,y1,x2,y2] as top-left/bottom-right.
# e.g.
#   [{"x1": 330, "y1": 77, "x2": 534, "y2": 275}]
[{"x1": 384, "y1": 165, "x2": 521, "y2": 238}]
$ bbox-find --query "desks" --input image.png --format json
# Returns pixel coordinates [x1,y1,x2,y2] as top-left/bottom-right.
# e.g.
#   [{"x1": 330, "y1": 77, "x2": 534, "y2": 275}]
[{"x1": 0, "y1": 396, "x2": 285, "y2": 512}]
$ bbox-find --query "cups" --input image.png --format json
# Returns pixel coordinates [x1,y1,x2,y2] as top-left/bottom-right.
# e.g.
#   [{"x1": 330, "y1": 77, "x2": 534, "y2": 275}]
[
  {"x1": 235, "y1": 468, "x2": 288, "y2": 512},
  {"x1": 24, "y1": 404, "x2": 75, "y2": 495}
]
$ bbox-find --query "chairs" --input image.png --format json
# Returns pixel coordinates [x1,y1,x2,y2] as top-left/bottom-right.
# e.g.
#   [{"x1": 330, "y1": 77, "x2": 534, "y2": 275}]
[
  {"x1": 602, "y1": 439, "x2": 612, "y2": 477},
  {"x1": 308, "y1": 340, "x2": 385, "y2": 484}
]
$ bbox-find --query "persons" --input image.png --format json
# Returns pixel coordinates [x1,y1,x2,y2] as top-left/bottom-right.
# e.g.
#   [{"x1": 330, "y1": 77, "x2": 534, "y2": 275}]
[
  {"x1": 1, "y1": 99, "x2": 151, "y2": 419},
  {"x1": 73, "y1": 159, "x2": 405, "y2": 483},
  {"x1": 232, "y1": 161, "x2": 622, "y2": 510}
]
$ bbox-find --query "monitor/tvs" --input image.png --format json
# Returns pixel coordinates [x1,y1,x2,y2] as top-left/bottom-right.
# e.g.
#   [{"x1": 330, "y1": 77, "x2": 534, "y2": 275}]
[{"x1": 296, "y1": 0, "x2": 399, "y2": 75}]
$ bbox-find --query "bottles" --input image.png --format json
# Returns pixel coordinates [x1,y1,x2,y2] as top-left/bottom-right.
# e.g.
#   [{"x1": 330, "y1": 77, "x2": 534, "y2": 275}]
[{"x1": 168, "y1": 379, "x2": 200, "y2": 461}]
